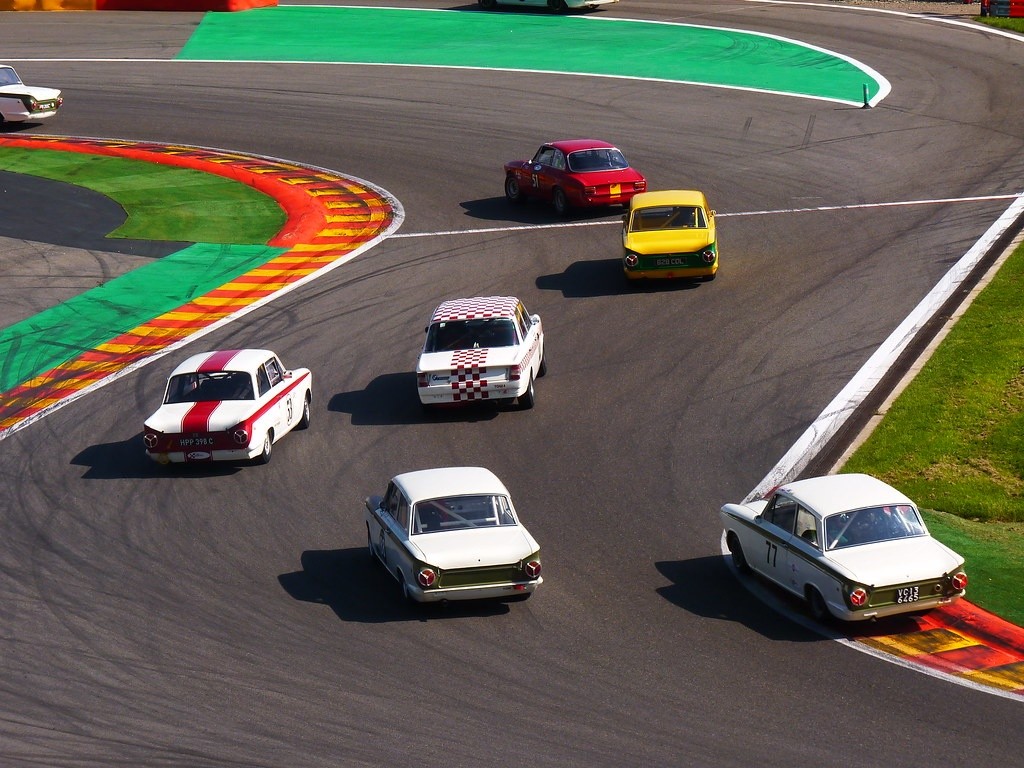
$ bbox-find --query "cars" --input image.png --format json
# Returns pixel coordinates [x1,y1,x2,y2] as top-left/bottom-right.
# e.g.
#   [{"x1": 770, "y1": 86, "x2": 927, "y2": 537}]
[
  {"x1": 364, "y1": 467, "x2": 544, "y2": 605},
  {"x1": 144, "y1": 350, "x2": 313, "y2": 464},
  {"x1": 417, "y1": 298, "x2": 547, "y2": 410},
  {"x1": 622, "y1": 190, "x2": 719, "y2": 282},
  {"x1": 1, "y1": 65, "x2": 63, "y2": 123},
  {"x1": 720, "y1": 472, "x2": 967, "y2": 625},
  {"x1": 477, "y1": 0, "x2": 618, "y2": 12},
  {"x1": 505, "y1": 140, "x2": 649, "y2": 217}
]
